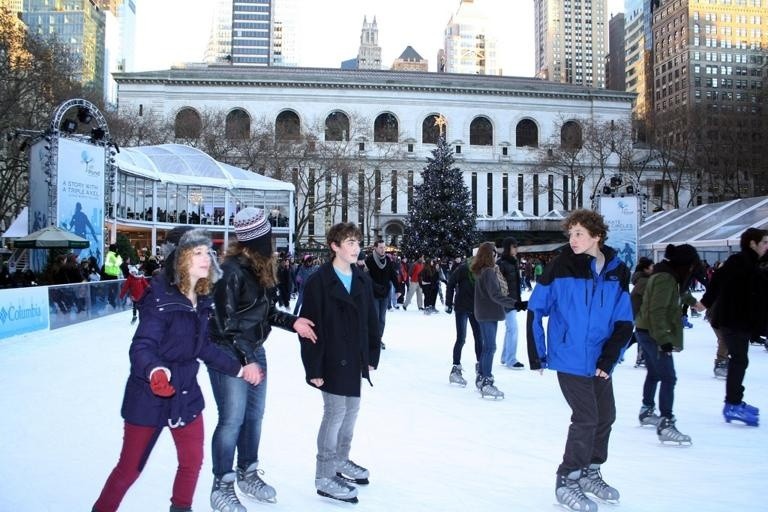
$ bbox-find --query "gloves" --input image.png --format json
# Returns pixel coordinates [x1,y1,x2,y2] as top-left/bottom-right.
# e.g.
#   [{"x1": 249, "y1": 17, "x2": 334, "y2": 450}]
[
  {"x1": 149, "y1": 369, "x2": 176, "y2": 397},
  {"x1": 660, "y1": 341, "x2": 673, "y2": 352},
  {"x1": 514, "y1": 300, "x2": 528, "y2": 311},
  {"x1": 445, "y1": 306, "x2": 452, "y2": 314}
]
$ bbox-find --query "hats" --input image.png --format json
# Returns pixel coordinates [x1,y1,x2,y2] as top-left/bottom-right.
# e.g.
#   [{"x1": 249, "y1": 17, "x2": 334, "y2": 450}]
[
  {"x1": 160, "y1": 226, "x2": 224, "y2": 286},
  {"x1": 639, "y1": 257, "x2": 654, "y2": 271},
  {"x1": 665, "y1": 244, "x2": 697, "y2": 265},
  {"x1": 503, "y1": 237, "x2": 520, "y2": 250},
  {"x1": 304, "y1": 255, "x2": 311, "y2": 261},
  {"x1": 233, "y1": 207, "x2": 272, "y2": 259},
  {"x1": 127, "y1": 264, "x2": 140, "y2": 274}
]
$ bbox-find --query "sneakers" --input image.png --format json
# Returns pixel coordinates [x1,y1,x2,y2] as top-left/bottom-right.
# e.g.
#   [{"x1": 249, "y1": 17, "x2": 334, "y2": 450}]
[
  {"x1": 749, "y1": 336, "x2": 765, "y2": 342},
  {"x1": 714, "y1": 358, "x2": 729, "y2": 377},
  {"x1": 682, "y1": 316, "x2": 693, "y2": 328},
  {"x1": 639, "y1": 406, "x2": 660, "y2": 426},
  {"x1": 234, "y1": 460, "x2": 277, "y2": 500},
  {"x1": 581, "y1": 463, "x2": 620, "y2": 500},
  {"x1": 636, "y1": 343, "x2": 647, "y2": 365},
  {"x1": 657, "y1": 417, "x2": 691, "y2": 442},
  {"x1": 691, "y1": 310, "x2": 702, "y2": 317},
  {"x1": 393, "y1": 304, "x2": 399, "y2": 309},
  {"x1": 425, "y1": 305, "x2": 438, "y2": 313},
  {"x1": 315, "y1": 476, "x2": 359, "y2": 499},
  {"x1": 419, "y1": 307, "x2": 424, "y2": 311},
  {"x1": 723, "y1": 403, "x2": 759, "y2": 424},
  {"x1": 291, "y1": 293, "x2": 296, "y2": 298},
  {"x1": 481, "y1": 376, "x2": 504, "y2": 396},
  {"x1": 555, "y1": 461, "x2": 598, "y2": 512},
  {"x1": 448, "y1": 364, "x2": 467, "y2": 386},
  {"x1": 209, "y1": 471, "x2": 248, "y2": 512},
  {"x1": 511, "y1": 362, "x2": 525, "y2": 370},
  {"x1": 336, "y1": 460, "x2": 370, "y2": 479},
  {"x1": 740, "y1": 401, "x2": 758, "y2": 415},
  {"x1": 131, "y1": 317, "x2": 136, "y2": 324},
  {"x1": 403, "y1": 305, "x2": 406, "y2": 309}
]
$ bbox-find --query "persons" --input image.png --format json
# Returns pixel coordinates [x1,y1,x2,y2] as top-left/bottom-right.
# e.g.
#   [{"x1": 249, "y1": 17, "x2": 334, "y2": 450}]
[{"x1": 1, "y1": 201, "x2": 768, "y2": 512}]
